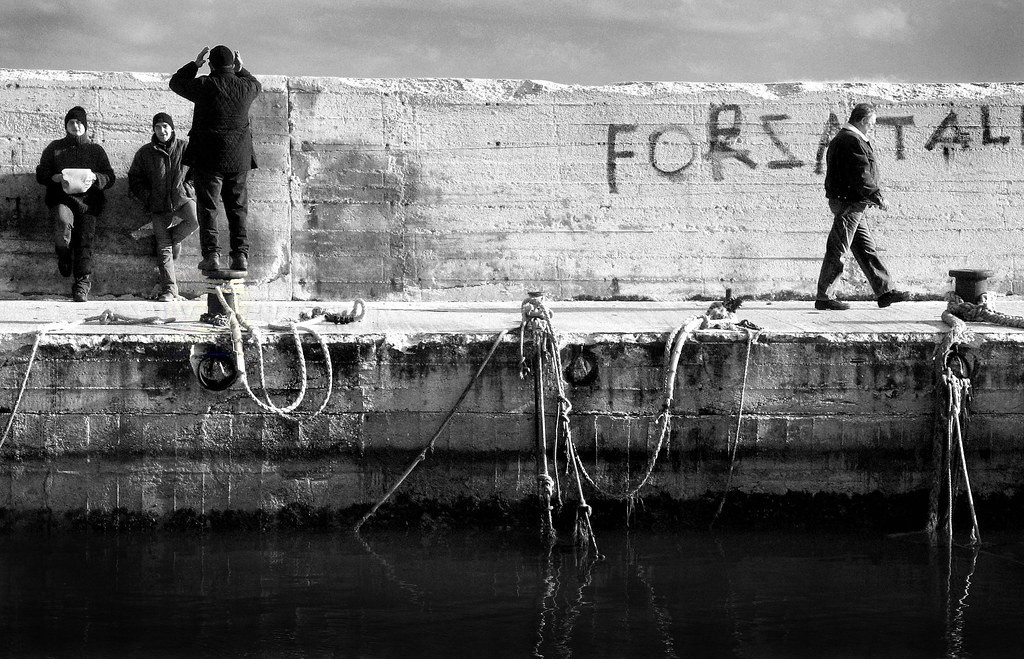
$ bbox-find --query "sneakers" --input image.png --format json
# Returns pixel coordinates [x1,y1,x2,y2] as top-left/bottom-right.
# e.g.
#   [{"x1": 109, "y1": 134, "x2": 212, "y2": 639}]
[
  {"x1": 878, "y1": 290, "x2": 910, "y2": 308},
  {"x1": 815, "y1": 297, "x2": 850, "y2": 311},
  {"x1": 198, "y1": 252, "x2": 219, "y2": 270},
  {"x1": 230, "y1": 253, "x2": 248, "y2": 271}
]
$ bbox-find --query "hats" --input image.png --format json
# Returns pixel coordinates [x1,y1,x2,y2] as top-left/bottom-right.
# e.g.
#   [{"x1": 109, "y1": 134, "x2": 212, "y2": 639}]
[
  {"x1": 64, "y1": 105, "x2": 87, "y2": 132},
  {"x1": 153, "y1": 113, "x2": 174, "y2": 129},
  {"x1": 207, "y1": 46, "x2": 234, "y2": 66}
]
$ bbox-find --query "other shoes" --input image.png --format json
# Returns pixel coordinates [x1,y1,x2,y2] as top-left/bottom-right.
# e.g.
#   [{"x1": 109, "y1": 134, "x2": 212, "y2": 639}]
[
  {"x1": 58, "y1": 254, "x2": 72, "y2": 278},
  {"x1": 159, "y1": 286, "x2": 179, "y2": 301},
  {"x1": 170, "y1": 226, "x2": 181, "y2": 259},
  {"x1": 72, "y1": 280, "x2": 90, "y2": 300}
]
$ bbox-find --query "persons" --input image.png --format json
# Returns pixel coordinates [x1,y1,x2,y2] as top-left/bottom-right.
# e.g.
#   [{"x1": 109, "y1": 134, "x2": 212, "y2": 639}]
[
  {"x1": 169, "y1": 44, "x2": 262, "y2": 270},
  {"x1": 815, "y1": 103, "x2": 910, "y2": 310},
  {"x1": 36, "y1": 106, "x2": 115, "y2": 301},
  {"x1": 128, "y1": 112, "x2": 198, "y2": 301}
]
